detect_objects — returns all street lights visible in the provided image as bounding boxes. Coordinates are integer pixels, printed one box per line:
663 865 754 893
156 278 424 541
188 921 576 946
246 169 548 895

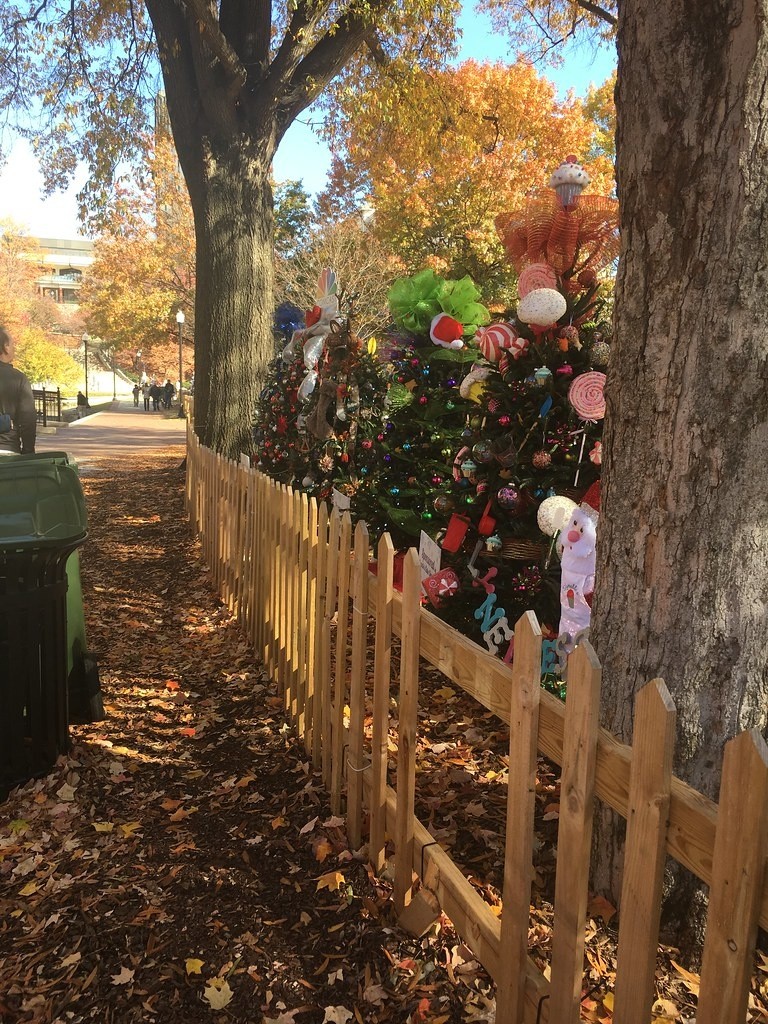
175 310 185 417
551 155 590 299
82 332 89 401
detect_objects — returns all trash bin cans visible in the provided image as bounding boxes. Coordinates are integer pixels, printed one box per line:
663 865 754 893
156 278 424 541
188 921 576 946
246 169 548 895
0 450 107 806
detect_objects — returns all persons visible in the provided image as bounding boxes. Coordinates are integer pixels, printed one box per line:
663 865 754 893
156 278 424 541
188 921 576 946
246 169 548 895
0 326 38 456
77 391 87 418
132 384 140 407
165 379 174 409
149 381 162 411
142 382 151 411
